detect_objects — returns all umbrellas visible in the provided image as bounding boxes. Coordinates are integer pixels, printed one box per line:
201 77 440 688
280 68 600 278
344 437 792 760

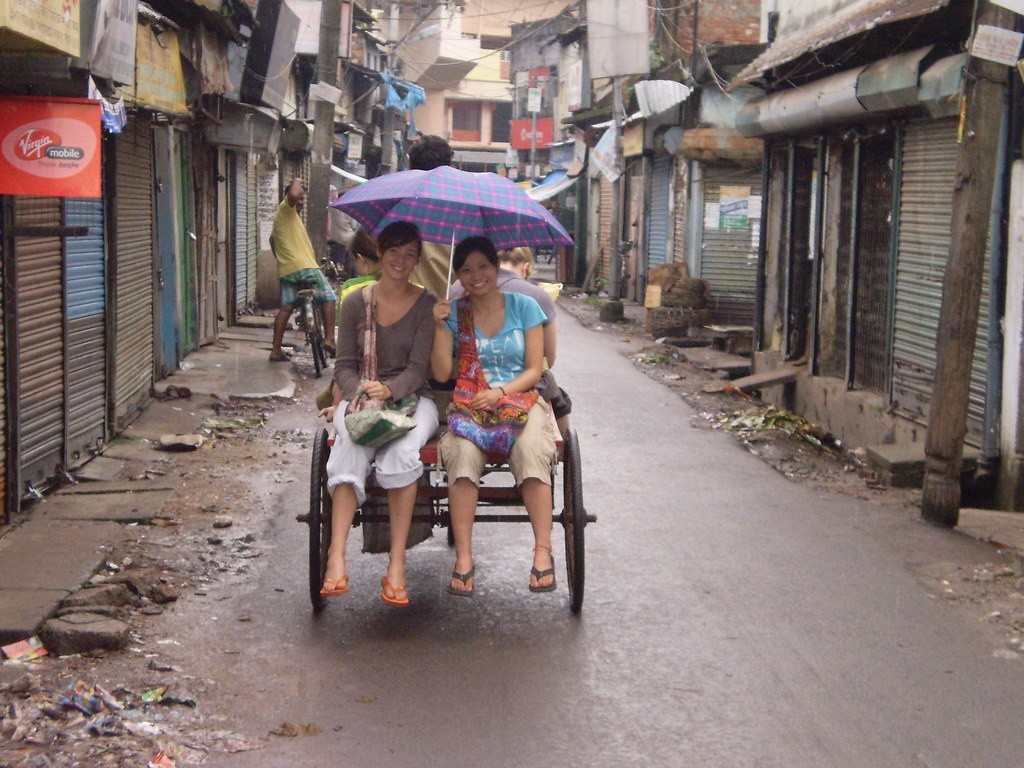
325 165 574 319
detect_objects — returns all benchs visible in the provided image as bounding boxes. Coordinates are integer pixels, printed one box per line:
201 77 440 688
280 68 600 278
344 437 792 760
327 361 563 452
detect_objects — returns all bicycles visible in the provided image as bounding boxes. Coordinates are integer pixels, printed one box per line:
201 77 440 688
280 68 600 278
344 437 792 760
295 257 339 380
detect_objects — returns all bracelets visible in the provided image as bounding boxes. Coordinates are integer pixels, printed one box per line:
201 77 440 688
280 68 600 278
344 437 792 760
498 387 505 395
333 402 337 403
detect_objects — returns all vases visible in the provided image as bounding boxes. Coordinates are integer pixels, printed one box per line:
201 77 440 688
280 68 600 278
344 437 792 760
644 284 700 309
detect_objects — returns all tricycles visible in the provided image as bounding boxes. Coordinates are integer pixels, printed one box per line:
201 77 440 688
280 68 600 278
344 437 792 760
297 369 598 619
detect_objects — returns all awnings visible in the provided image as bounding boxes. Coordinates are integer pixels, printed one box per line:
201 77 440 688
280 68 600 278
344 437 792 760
527 177 579 200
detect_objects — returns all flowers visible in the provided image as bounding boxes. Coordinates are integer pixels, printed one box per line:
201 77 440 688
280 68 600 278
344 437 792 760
649 263 711 300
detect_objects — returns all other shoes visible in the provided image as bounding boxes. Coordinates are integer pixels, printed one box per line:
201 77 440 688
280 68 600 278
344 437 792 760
269 352 290 361
320 341 335 359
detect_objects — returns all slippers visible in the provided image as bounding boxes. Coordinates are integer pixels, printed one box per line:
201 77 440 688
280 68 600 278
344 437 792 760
448 562 476 597
380 576 409 606
528 555 557 592
320 573 350 596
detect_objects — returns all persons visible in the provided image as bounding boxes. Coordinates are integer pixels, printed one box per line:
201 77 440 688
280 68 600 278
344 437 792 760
410 135 457 298
269 178 337 362
321 221 437 604
327 192 357 280
450 244 572 440
430 236 558 593
551 202 569 229
316 226 379 424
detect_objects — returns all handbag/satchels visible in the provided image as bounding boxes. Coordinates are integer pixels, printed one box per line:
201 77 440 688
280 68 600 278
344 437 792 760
343 390 416 448
446 379 538 458
